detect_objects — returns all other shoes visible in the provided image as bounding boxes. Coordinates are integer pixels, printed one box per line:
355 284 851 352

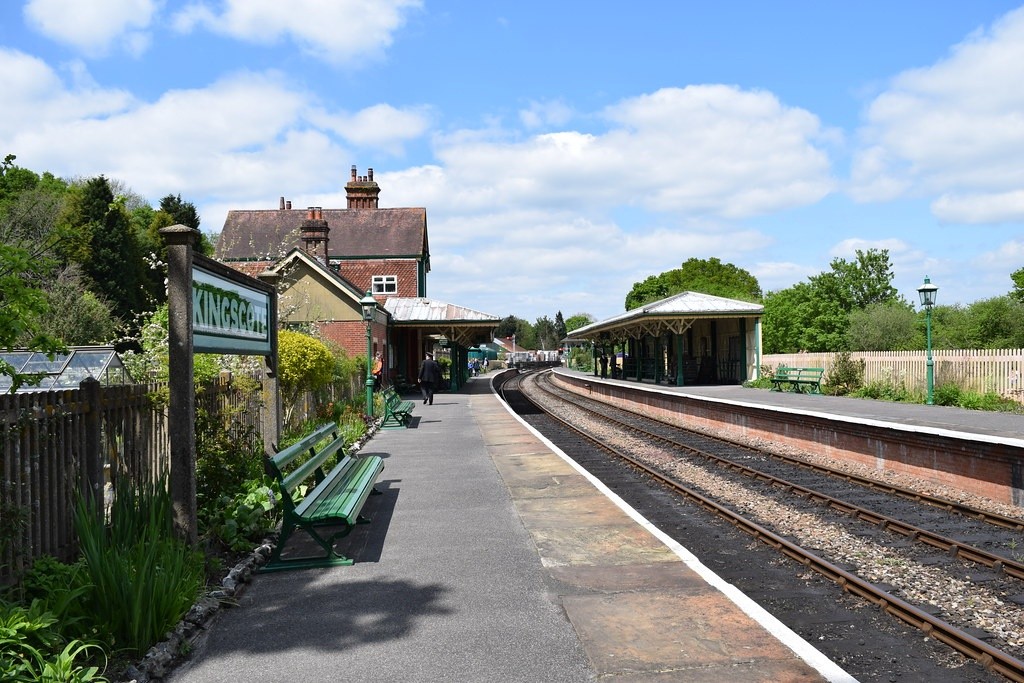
429 403 432 405
423 396 428 405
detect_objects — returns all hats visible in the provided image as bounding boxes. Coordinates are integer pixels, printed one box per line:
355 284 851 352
426 351 434 357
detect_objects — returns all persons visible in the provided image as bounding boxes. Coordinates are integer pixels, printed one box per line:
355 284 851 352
609 352 617 379
371 354 383 392
417 352 440 405
468 357 489 377
599 354 608 379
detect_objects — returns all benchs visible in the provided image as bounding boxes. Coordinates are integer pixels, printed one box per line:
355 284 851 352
379 385 415 429
770 367 824 394
257 421 384 572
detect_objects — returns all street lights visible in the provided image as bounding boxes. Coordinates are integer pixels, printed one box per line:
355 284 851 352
358 289 380 426
915 274 939 406
